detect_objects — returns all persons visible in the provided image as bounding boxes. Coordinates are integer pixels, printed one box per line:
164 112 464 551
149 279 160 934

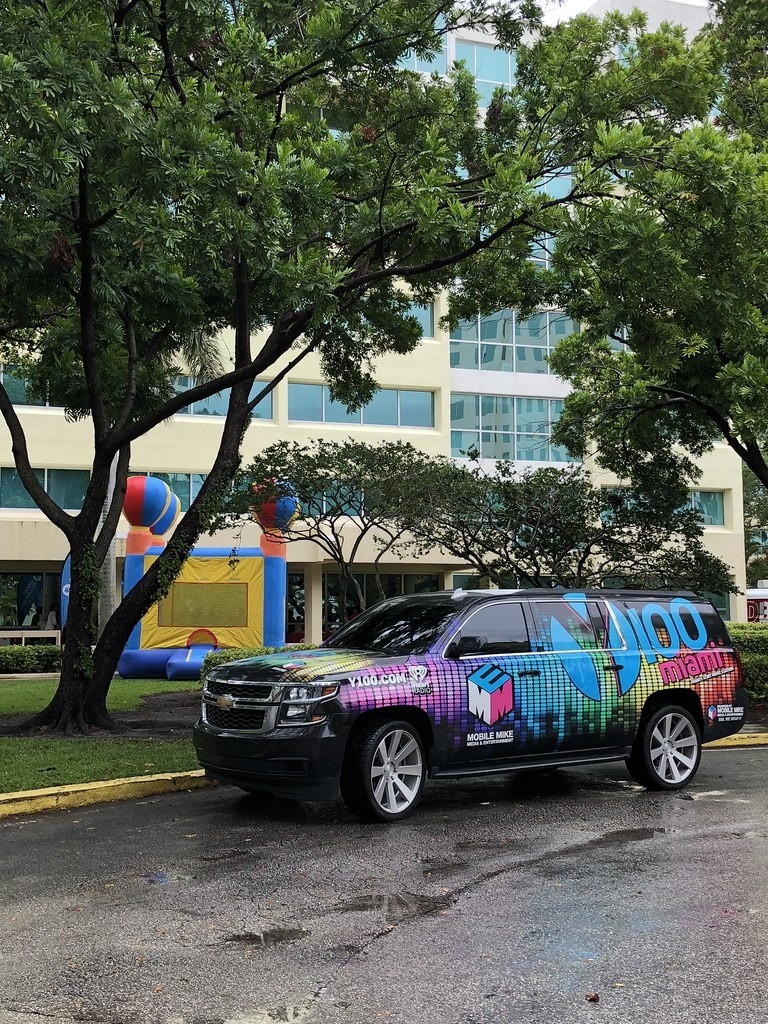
45 603 59 644
31 606 43 627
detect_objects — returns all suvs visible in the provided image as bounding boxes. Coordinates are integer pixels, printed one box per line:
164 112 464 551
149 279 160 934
192 586 750 824
